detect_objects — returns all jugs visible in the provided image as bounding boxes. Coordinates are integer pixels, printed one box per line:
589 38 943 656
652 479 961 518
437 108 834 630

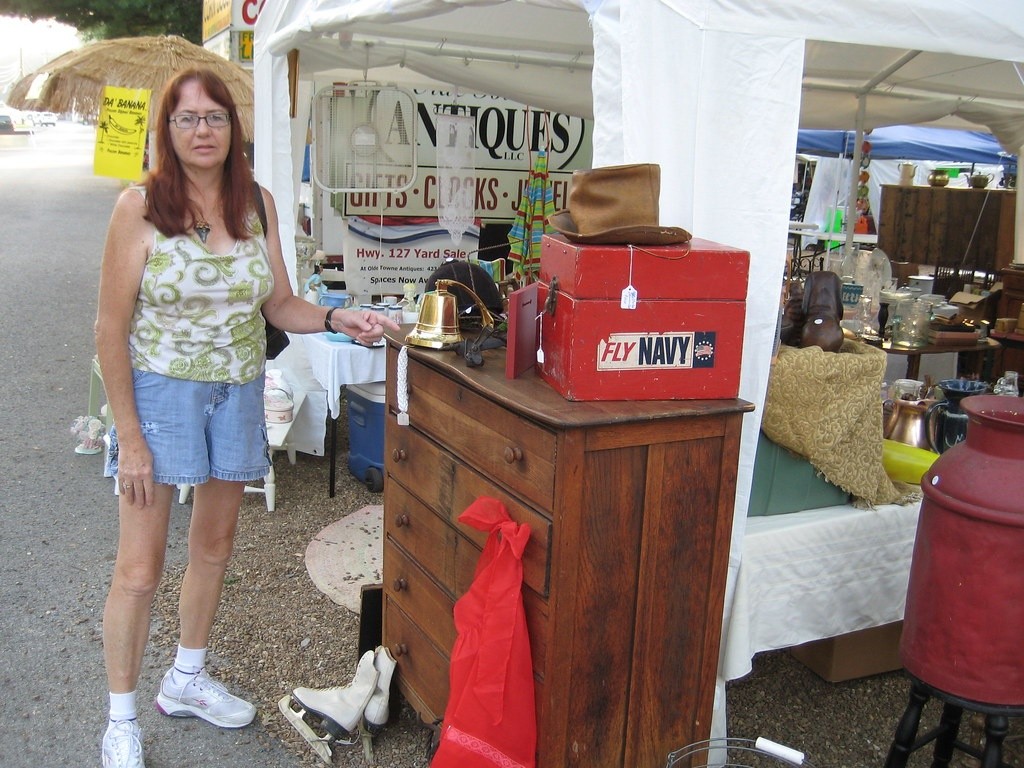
878 371 1019 457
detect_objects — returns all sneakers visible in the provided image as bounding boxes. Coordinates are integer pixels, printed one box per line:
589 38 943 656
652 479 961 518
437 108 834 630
155 664 257 729
102 718 146 768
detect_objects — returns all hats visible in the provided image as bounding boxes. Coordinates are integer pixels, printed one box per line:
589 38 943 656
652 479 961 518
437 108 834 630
547 163 692 246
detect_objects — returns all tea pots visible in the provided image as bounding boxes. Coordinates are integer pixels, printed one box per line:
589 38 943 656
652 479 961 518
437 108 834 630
898 160 918 187
964 171 995 189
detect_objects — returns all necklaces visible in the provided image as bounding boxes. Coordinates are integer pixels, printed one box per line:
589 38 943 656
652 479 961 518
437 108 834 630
191 200 220 243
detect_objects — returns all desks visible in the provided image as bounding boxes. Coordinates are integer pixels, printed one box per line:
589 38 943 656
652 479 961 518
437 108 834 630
855 332 1002 380
178 394 308 512
787 230 877 283
381 318 755 768
709 485 925 768
286 331 386 496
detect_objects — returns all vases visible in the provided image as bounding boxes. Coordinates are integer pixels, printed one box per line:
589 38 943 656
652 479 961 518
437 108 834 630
926 380 986 457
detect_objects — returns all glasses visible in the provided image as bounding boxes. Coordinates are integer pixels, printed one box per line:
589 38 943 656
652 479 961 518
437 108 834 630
168 113 230 129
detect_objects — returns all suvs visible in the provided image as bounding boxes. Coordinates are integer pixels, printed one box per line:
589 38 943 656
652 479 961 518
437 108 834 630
0 116 15 133
32 112 59 126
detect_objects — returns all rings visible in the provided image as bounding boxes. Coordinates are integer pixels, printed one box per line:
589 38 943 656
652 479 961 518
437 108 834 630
123 484 131 488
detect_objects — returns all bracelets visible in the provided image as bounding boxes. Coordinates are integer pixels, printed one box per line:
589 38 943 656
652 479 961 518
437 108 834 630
325 306 343 334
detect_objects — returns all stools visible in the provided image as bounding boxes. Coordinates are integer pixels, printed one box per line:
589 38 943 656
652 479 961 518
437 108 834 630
89 354 112 478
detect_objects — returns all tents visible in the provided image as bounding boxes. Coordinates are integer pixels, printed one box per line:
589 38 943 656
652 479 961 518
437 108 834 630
265 1 1024 276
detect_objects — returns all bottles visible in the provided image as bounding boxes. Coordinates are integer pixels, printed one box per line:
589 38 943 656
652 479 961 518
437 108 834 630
854 276 959 350
896 396 1024 707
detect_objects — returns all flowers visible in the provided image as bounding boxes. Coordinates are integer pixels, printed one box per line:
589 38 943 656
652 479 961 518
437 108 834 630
69 415 106 454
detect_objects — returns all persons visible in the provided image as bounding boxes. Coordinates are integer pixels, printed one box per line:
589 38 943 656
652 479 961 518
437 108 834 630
99 67 402 768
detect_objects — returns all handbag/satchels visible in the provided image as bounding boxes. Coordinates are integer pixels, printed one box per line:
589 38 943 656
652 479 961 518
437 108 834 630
250 179 290 361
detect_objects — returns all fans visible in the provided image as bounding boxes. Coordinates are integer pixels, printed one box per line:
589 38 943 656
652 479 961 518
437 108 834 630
312 85 418 193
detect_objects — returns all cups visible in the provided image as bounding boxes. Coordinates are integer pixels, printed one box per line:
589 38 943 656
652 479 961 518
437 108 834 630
928 168 949 186
360 297 403 324
994 318 1018 334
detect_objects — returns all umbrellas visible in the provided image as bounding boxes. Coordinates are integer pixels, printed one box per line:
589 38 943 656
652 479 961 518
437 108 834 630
1 34 262 171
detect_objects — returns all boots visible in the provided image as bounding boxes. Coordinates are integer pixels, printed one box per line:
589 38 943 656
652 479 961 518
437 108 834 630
358 645 397 763
278 650 380 764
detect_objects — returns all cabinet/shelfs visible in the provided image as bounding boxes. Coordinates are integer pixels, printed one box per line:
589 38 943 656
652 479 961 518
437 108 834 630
998 266 1024 324
879 184 1017 271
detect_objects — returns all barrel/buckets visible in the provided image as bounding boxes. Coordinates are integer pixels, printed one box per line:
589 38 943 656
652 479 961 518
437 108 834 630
263 386 295 447
319 292 352 308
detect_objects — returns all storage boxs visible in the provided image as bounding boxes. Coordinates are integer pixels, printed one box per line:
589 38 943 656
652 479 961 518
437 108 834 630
783 619 907 683
341 381 387 492
947 281 1004 325
537 234 748 400
